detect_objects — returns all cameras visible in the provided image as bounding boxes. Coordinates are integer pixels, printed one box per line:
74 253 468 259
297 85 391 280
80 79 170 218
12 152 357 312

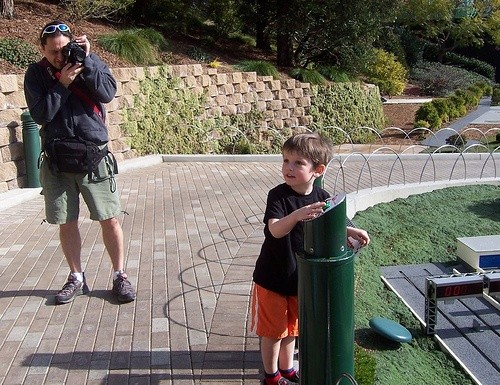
62 40 86 68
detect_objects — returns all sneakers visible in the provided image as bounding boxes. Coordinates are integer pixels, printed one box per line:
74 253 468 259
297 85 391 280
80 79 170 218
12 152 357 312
54 272 90 303
112 271 136 303
263 369 299 385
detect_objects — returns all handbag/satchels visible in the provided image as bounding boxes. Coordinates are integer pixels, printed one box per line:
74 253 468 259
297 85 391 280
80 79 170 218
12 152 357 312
51 136 100 173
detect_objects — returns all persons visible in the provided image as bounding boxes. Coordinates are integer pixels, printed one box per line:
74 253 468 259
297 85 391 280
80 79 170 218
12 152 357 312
23 19 137 304
250 132 370 385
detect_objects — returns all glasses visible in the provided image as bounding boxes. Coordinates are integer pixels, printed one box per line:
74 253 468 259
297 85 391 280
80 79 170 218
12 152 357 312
41 23 71 38
